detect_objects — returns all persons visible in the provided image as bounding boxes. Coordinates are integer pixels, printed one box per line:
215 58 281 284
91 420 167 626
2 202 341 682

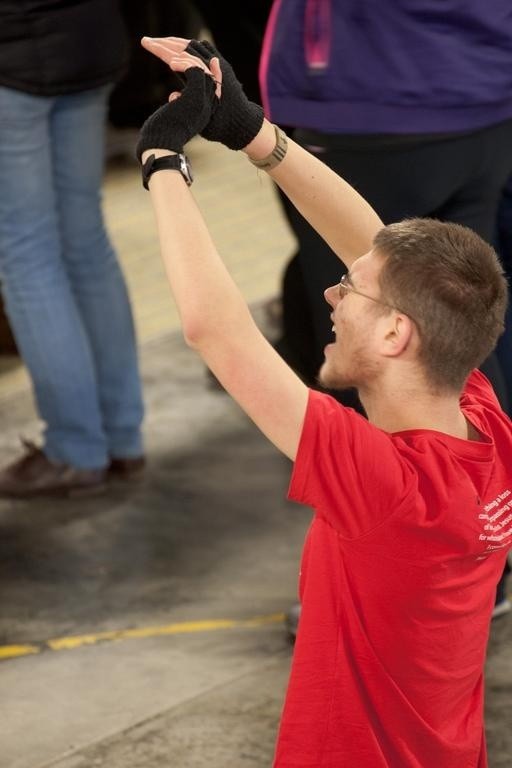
0 1 170 501
211 283 292 398
205 0 512 646
105 0 207 169
135 34 512 765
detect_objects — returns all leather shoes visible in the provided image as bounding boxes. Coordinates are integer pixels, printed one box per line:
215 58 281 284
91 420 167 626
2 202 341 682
1 442 143 500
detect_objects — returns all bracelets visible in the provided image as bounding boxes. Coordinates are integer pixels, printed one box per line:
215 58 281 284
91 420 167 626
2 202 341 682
247 121 287 172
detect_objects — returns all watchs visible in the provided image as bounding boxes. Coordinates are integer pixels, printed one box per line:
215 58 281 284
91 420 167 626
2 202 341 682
140 152 193 190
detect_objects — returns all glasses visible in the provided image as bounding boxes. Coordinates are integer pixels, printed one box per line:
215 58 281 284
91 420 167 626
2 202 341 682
339 274 423 337
